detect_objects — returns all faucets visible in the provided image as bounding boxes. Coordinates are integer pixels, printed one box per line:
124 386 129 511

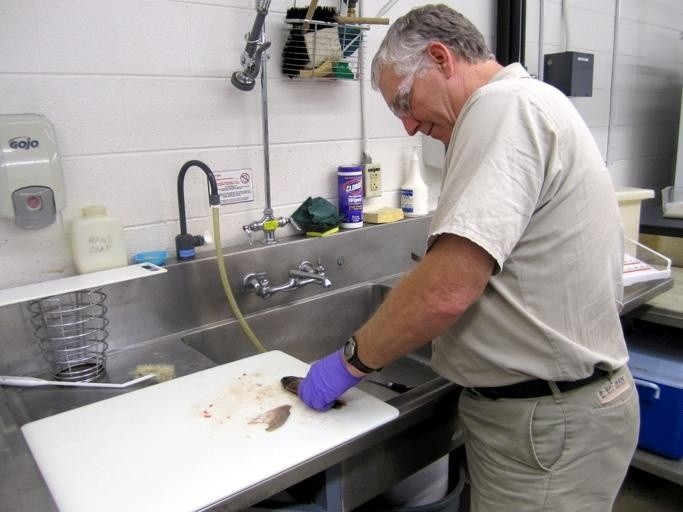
289 270 332 289
174 159 220 260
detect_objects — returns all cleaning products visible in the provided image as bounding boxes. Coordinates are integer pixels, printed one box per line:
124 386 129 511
400 144 428 217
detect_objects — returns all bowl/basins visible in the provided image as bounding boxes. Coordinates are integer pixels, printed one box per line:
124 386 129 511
401 458 467 511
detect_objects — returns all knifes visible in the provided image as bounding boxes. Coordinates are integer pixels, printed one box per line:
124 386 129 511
363 369 416 394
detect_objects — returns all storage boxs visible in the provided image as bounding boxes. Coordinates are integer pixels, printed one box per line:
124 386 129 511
623 327 683 460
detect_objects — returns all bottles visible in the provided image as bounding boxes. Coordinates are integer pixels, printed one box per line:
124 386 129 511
337 164 364 230
71 203 128 275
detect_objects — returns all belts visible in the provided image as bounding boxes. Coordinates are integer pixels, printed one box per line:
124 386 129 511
465 365 620 400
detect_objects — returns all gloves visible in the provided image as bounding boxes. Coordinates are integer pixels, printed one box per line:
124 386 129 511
296 349 366 413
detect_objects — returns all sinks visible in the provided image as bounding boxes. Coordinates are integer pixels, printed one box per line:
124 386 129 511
181 284 442 402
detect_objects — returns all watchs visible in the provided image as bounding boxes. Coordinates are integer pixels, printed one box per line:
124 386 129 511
341 334 385 374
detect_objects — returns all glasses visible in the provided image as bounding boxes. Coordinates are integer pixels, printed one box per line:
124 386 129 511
389 48 429 117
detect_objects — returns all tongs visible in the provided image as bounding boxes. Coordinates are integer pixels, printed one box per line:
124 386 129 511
22 347 400 512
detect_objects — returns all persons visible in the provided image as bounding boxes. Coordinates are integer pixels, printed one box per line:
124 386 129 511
297 2 643 512
